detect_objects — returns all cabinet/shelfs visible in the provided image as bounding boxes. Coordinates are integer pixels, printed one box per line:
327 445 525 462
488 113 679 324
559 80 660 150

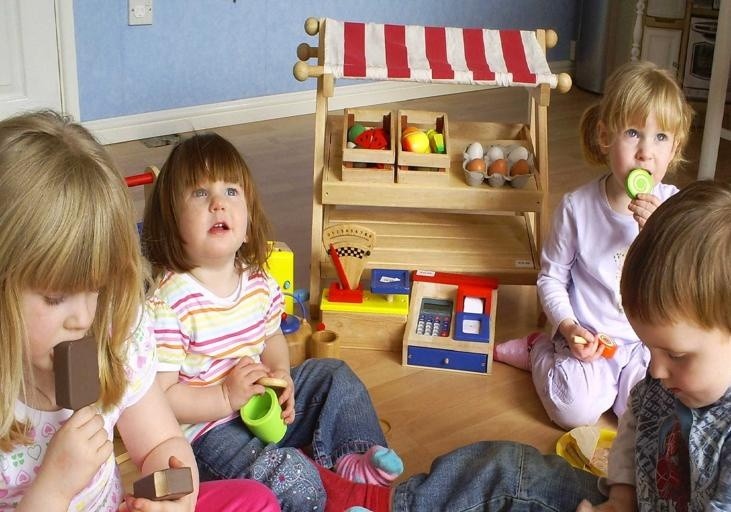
603 0 731 102
294 19 572 327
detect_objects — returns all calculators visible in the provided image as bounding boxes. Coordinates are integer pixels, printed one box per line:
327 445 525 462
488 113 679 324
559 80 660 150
416 298 452 337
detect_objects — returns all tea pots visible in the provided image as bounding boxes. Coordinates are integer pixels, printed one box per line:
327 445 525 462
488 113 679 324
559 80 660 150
277 292 325 369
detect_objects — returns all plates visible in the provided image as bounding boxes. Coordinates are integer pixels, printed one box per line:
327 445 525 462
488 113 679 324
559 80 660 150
555 427 619 476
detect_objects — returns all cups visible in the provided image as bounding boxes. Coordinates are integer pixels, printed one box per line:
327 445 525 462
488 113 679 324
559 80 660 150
311 329 341 359
240 387 287 444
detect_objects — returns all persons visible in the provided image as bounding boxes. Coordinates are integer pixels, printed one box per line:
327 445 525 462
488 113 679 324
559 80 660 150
494 58 696 431
1 107 278 511
126 129 406 510
390 178 731 509
